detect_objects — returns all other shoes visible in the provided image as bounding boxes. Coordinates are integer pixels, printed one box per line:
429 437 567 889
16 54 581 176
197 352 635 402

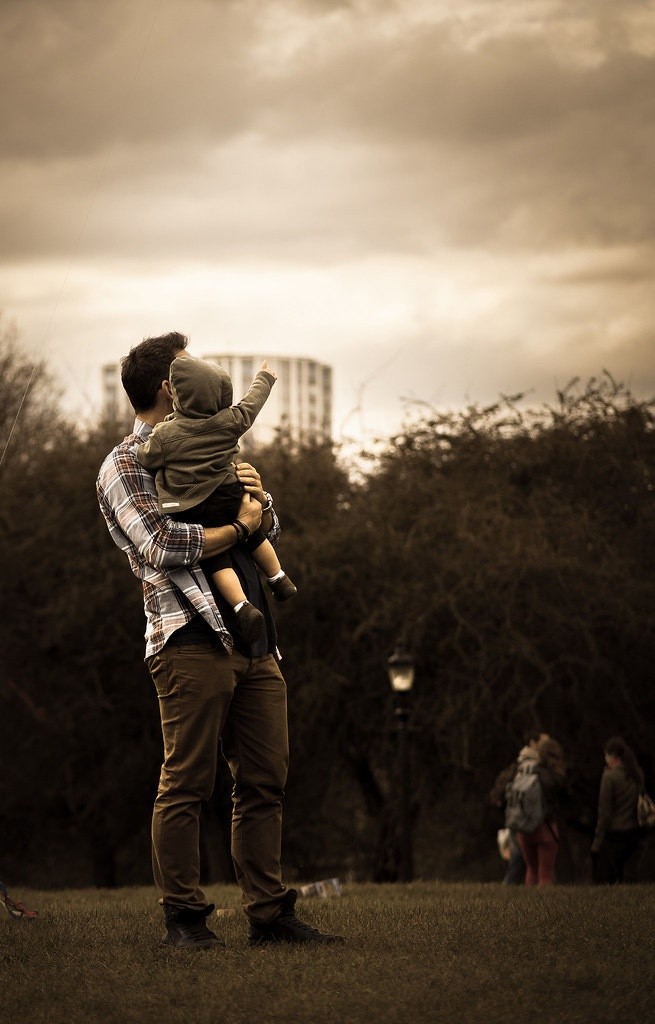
237 601 264 646
269 574 298 604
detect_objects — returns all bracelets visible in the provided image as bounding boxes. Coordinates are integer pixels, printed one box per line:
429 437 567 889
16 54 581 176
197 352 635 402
230 519 252 545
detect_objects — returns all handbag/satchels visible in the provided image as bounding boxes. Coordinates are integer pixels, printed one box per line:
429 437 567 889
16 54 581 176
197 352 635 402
497 828 518 860
638 789 655 827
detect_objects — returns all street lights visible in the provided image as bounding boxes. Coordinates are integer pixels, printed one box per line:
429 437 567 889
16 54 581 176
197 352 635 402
386 644 418 884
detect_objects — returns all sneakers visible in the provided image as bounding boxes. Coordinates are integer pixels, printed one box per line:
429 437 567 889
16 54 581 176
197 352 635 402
164 903 226 953
248 888 345 949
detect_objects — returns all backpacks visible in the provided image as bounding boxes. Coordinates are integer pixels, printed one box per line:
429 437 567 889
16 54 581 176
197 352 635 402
506 771 545 834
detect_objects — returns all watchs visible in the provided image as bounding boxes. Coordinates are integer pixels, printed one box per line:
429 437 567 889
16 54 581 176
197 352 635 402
262 491 274 515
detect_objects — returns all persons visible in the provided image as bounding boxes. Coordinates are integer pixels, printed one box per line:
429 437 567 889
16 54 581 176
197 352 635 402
95 332 345 950
137 354 298 645
591 736 646 886
491 731 565 886
